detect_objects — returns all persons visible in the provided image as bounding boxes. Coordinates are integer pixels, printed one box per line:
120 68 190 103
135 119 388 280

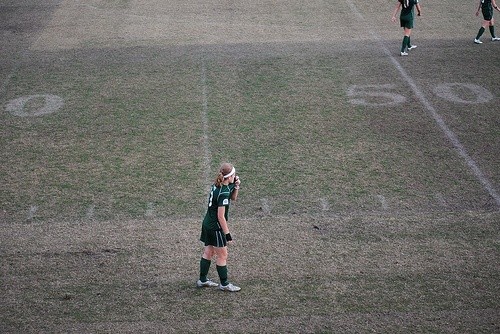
474 0 500 44
196 162 241 292
391 0 421 56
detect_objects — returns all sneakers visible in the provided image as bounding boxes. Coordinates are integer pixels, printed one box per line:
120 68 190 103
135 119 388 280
492 36 500 41
407 45 416 51
400 51 408 55
475 39 483 43
220 283 241 292
197 280 218 286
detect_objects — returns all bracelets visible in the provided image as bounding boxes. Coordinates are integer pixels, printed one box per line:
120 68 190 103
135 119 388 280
234 187 240 189
